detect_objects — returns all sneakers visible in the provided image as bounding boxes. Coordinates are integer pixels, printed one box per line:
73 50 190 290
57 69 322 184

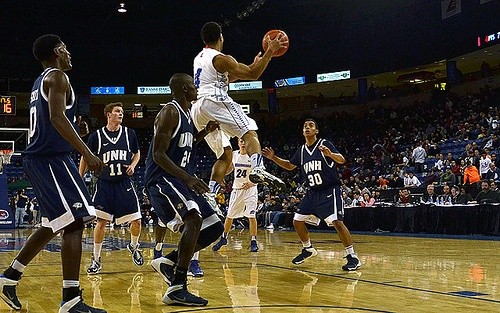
162 284 208 306
249 241 258 251
154 248 162 259
59 288 106 313
127 242 144 266
292 247 317 264
87 257 101 274
212 236 227 251
0 275 21 311
187 259 204 276
150 257 175 285
342 254 361 271
202 191 224 219
249 167 286 192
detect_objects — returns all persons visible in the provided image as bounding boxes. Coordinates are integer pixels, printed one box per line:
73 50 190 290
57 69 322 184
145 73 220 307
79 102 144 275
212 139 258 252
0 85 500 204
262 119 361 271
191 22 286 197
0 34 106 313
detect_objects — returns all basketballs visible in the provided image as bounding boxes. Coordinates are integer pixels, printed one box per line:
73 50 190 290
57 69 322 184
262 29 289 56
